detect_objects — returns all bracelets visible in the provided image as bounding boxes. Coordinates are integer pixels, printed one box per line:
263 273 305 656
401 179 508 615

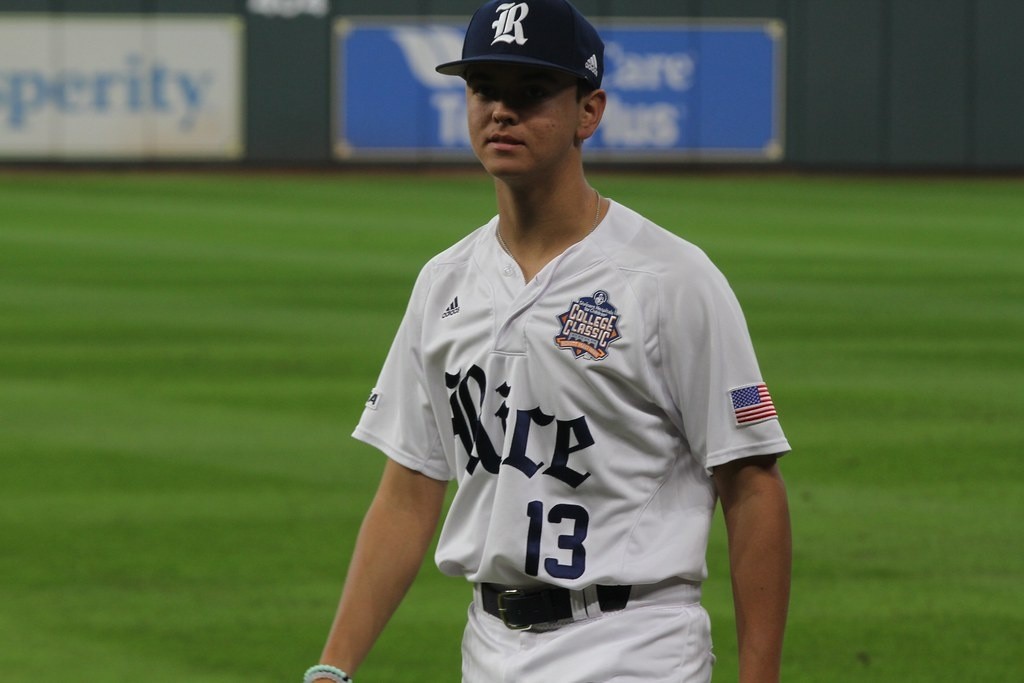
303 663 353 683
302 0 795 683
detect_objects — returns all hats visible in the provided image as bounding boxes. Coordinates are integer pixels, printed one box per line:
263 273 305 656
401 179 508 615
435 0 605 89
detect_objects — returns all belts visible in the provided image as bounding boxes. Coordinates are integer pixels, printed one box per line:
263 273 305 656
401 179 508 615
481 583 632 630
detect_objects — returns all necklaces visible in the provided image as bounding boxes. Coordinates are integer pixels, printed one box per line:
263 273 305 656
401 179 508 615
496 189 601 261
